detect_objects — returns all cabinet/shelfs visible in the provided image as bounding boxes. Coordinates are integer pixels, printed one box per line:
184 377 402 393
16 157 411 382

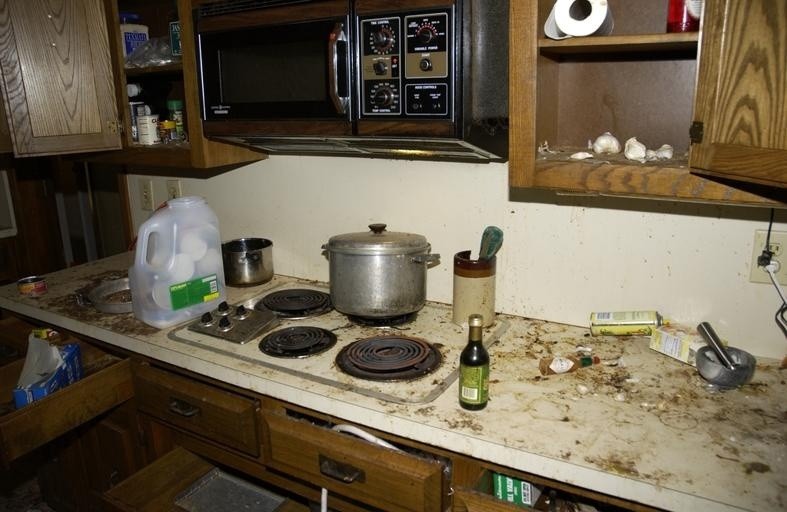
131 360 262 470
0 0 269 168
261 399 449 512
508 0 787 210
1 311 132 510
456 458 632 509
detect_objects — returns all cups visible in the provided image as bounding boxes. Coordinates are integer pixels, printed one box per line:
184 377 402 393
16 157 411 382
454 250 497 329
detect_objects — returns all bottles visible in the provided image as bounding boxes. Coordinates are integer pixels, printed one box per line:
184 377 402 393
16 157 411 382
459 314 491 410
166 88 185 134
588 309 674 337
667 0 701 31
166 0 184 66
118 13 149 56
539 355 602 374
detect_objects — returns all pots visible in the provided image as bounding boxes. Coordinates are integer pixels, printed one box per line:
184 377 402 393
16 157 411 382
321 222 439 319
220 238 274 286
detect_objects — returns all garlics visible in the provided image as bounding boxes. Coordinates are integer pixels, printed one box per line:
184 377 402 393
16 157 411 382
625 136 673 161
593 132 620 153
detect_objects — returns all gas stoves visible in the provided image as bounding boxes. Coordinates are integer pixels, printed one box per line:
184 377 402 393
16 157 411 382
167 276 512 406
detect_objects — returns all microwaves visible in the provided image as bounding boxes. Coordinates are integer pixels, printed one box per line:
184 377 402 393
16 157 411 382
191 0 510 121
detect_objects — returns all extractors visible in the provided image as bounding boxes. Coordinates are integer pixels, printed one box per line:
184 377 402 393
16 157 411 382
205 124 510 165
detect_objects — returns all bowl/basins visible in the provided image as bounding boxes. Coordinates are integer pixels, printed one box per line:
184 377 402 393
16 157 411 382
698 347 756 388
87 278 134 315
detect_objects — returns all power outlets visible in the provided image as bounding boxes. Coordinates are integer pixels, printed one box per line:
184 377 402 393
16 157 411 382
749 231 786 286
138 179 154 210
167 180 181 201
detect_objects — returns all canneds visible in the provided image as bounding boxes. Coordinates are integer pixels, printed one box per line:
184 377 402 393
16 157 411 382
159 120 177 145
17 276 48 296
